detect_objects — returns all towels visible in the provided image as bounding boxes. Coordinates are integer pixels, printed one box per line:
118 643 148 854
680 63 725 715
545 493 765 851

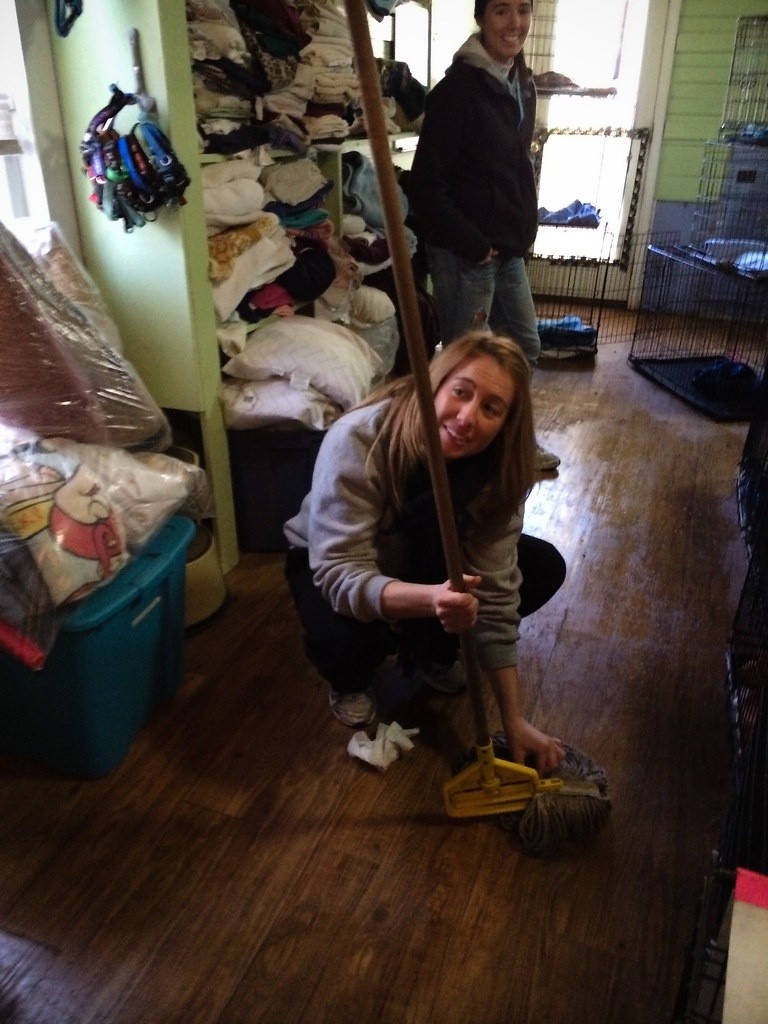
196 159 265 224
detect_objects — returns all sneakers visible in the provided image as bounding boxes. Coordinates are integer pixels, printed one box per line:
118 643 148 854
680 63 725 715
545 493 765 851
533 443 561 472
328 686 377 727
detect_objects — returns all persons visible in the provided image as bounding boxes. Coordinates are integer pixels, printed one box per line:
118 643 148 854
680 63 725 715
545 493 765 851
282 329 567 779
405 1 562 473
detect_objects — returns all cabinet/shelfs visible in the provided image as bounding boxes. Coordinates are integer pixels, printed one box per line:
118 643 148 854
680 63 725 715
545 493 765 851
200 124 420 357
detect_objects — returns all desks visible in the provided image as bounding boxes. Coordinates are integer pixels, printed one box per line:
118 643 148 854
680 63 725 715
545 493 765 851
692 867 768 1024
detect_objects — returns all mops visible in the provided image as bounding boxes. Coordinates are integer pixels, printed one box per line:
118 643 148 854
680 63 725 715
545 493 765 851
343 0 613 859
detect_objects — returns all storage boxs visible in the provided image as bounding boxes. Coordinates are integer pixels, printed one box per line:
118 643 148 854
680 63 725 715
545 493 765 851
36 514 198 777
